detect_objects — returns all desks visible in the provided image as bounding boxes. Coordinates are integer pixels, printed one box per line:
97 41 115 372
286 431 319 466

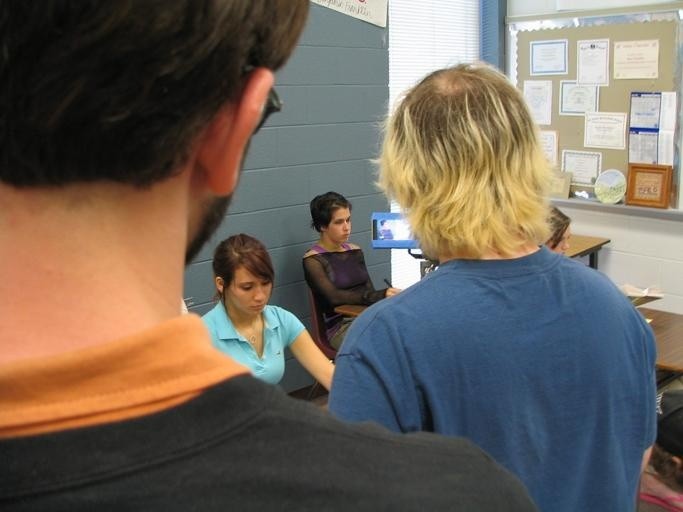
562 233 683 431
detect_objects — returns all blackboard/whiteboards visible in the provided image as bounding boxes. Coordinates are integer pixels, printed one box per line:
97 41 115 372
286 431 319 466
504 2 683 221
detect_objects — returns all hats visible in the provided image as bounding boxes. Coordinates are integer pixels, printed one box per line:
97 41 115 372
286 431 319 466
656 388 683 458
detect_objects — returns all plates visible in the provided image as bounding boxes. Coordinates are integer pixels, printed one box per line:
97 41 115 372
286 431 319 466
595 169 627 204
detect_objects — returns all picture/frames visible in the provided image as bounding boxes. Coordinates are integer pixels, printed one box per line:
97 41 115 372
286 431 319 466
625 162 673 209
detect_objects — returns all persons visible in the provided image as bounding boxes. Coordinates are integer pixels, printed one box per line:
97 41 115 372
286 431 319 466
328 61 658 512
639 388 683 510
0 0 540 512
303 191 402 352
201 233 335 392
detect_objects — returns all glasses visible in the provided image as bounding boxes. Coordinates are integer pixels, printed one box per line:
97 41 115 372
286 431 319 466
232 62 282 136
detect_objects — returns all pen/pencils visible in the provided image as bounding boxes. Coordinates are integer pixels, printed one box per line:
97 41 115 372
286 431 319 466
384 279 393 288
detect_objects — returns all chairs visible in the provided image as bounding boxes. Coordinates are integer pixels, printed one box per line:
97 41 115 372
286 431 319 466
304 284 339 403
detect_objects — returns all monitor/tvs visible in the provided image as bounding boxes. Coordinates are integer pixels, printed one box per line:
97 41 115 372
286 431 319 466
371 213 420 249
